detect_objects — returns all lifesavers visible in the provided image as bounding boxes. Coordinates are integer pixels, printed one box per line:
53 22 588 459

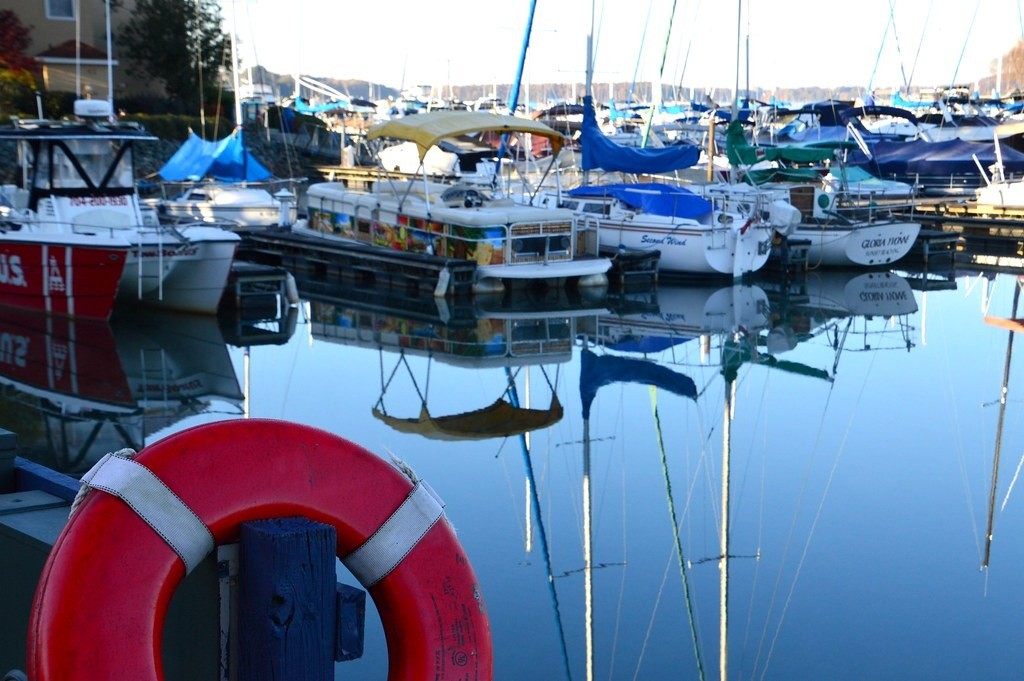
25 417 493 681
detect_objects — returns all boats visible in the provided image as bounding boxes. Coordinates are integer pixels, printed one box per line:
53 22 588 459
0 303 255 501
224 295 300 353
0 3 1024 317
762 266 922 386
284 268 609 441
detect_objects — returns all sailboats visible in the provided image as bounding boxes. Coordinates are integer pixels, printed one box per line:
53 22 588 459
578 275 773 681
